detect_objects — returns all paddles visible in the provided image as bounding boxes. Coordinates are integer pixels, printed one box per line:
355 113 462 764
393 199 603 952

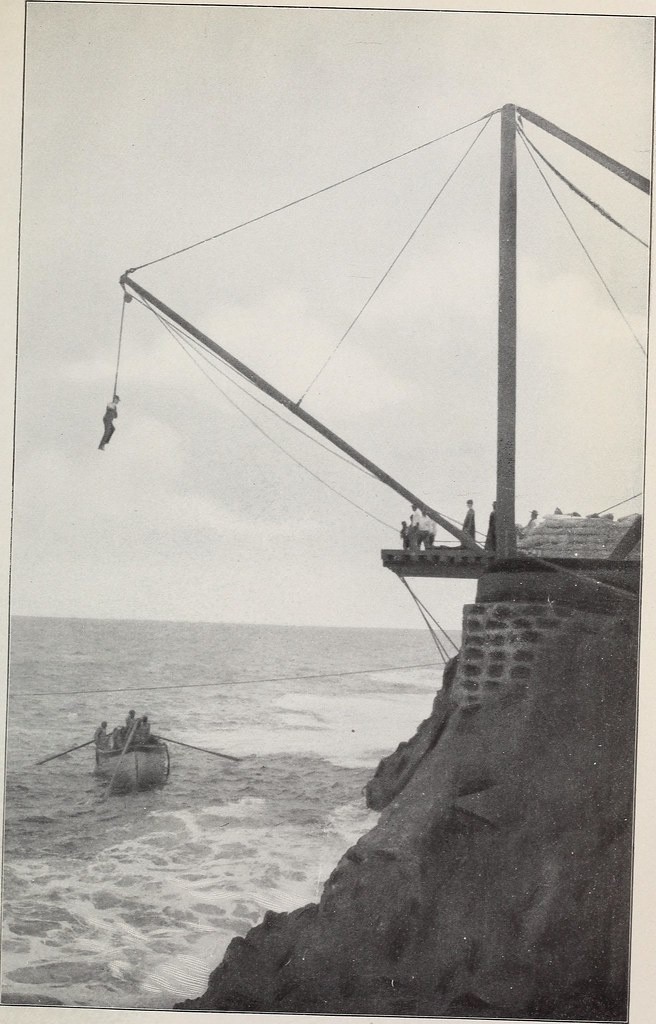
24 737 97 769
101 718 142 797
150 732 243 762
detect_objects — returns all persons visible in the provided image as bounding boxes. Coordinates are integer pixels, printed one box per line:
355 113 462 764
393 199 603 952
94 710 151 751
522 509 540 528
400 504 437 550
484 501 497 552
462 500 475 541
98 395 121 451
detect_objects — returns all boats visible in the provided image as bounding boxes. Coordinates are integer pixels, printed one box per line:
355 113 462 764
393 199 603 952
94 717 170 798
